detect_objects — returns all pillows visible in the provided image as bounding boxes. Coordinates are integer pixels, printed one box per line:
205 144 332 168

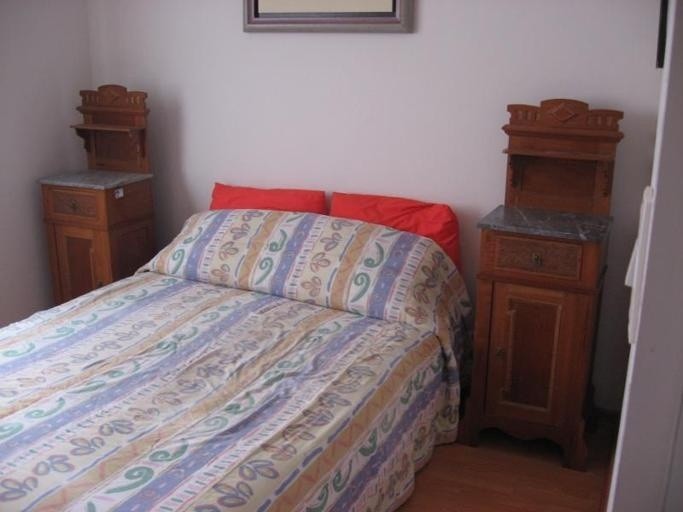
210 182 462 273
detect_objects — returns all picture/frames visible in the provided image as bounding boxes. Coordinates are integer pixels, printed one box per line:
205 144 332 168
241 0 414 34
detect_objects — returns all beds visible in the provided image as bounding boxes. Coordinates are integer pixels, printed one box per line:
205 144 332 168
0 211 473 512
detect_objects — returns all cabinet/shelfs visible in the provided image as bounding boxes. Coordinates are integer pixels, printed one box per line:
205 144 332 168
458 98 627 474
34 84 159 306
